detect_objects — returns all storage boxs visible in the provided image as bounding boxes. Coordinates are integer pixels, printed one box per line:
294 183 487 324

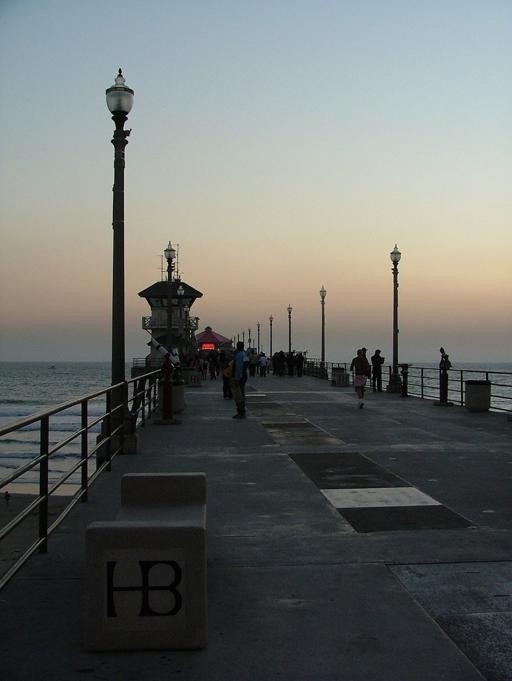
87 470 207 651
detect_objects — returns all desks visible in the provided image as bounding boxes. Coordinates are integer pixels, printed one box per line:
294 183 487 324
164 241 185 352
105 67 134 381
233 328 250 347
256 321 260 354
287 304 292 352
389 243 402 373
268 314 273 356
319 285 326 361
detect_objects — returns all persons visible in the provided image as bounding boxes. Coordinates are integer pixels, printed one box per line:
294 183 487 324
369 350 384 395
355 348 370 393
228 342 248 421
221 349 232 401
350 349 370 410
184 348 308 379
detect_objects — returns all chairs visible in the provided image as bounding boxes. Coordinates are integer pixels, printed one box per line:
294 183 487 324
231 410 247 419
358 401 364 409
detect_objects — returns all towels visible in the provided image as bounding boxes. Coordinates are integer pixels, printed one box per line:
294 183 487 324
159 380 185 414
332 367 344 386
465 380 491 412
182 367 198 385
